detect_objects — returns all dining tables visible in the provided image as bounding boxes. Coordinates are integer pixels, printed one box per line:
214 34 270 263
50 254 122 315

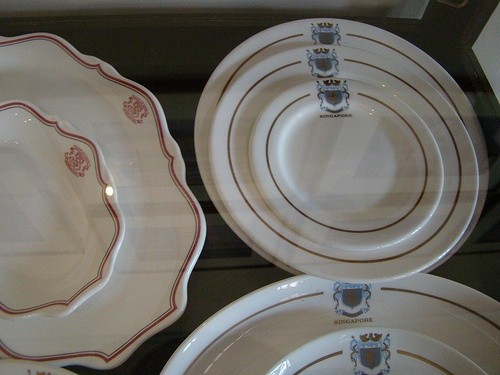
0 4 500 375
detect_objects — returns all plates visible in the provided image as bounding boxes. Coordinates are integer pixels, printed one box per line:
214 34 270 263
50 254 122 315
1 32 207 370
0 100 126 319
263 326 491 375
154 275 500 375
193 14 489 284
